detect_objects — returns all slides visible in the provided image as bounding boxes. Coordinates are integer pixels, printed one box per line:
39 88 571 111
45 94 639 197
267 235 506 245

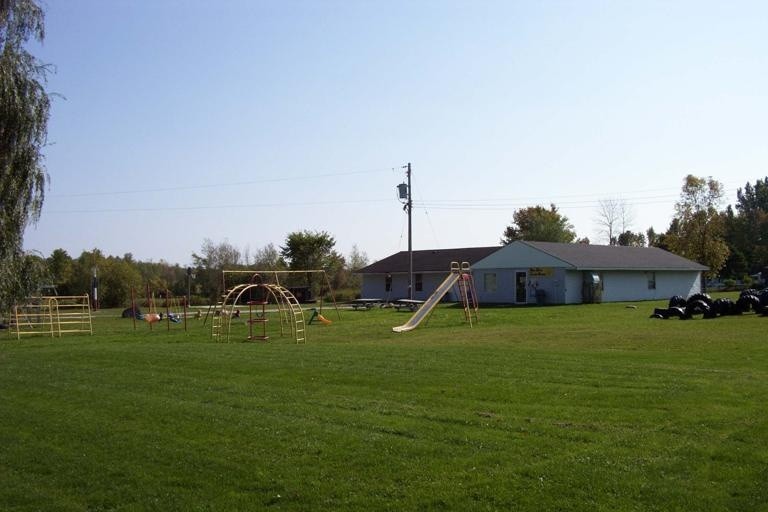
391 274 460 333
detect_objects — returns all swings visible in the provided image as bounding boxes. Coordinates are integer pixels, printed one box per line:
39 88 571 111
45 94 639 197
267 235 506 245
134 285 183 326
